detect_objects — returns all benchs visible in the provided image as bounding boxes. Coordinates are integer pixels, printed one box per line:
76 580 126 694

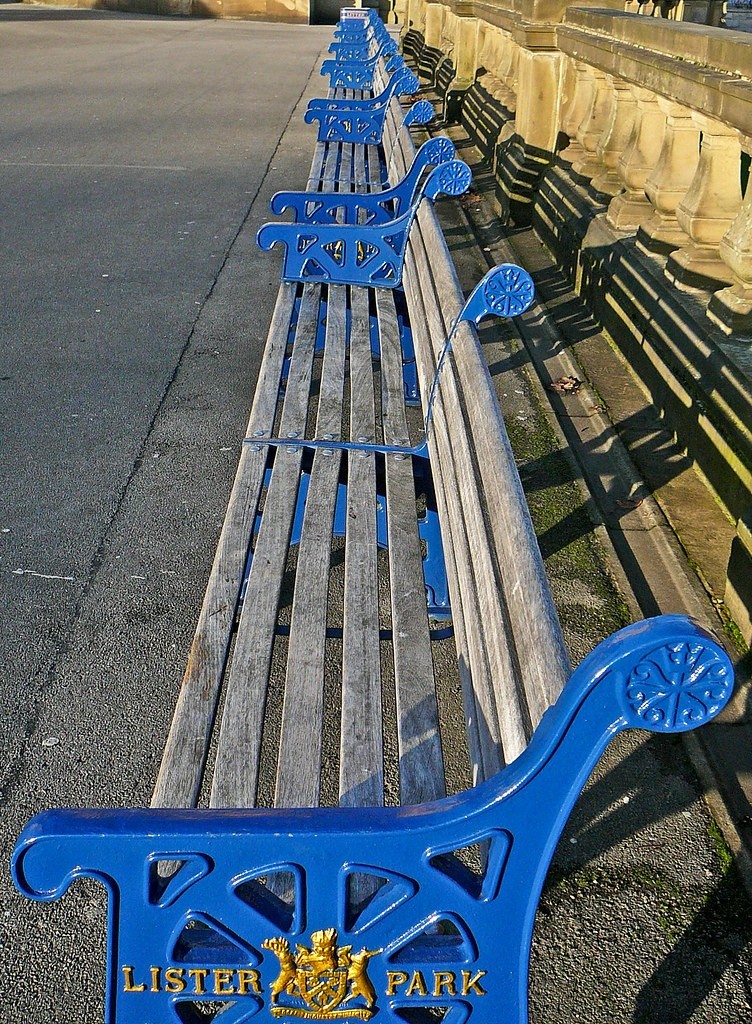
11 8 735 1024
